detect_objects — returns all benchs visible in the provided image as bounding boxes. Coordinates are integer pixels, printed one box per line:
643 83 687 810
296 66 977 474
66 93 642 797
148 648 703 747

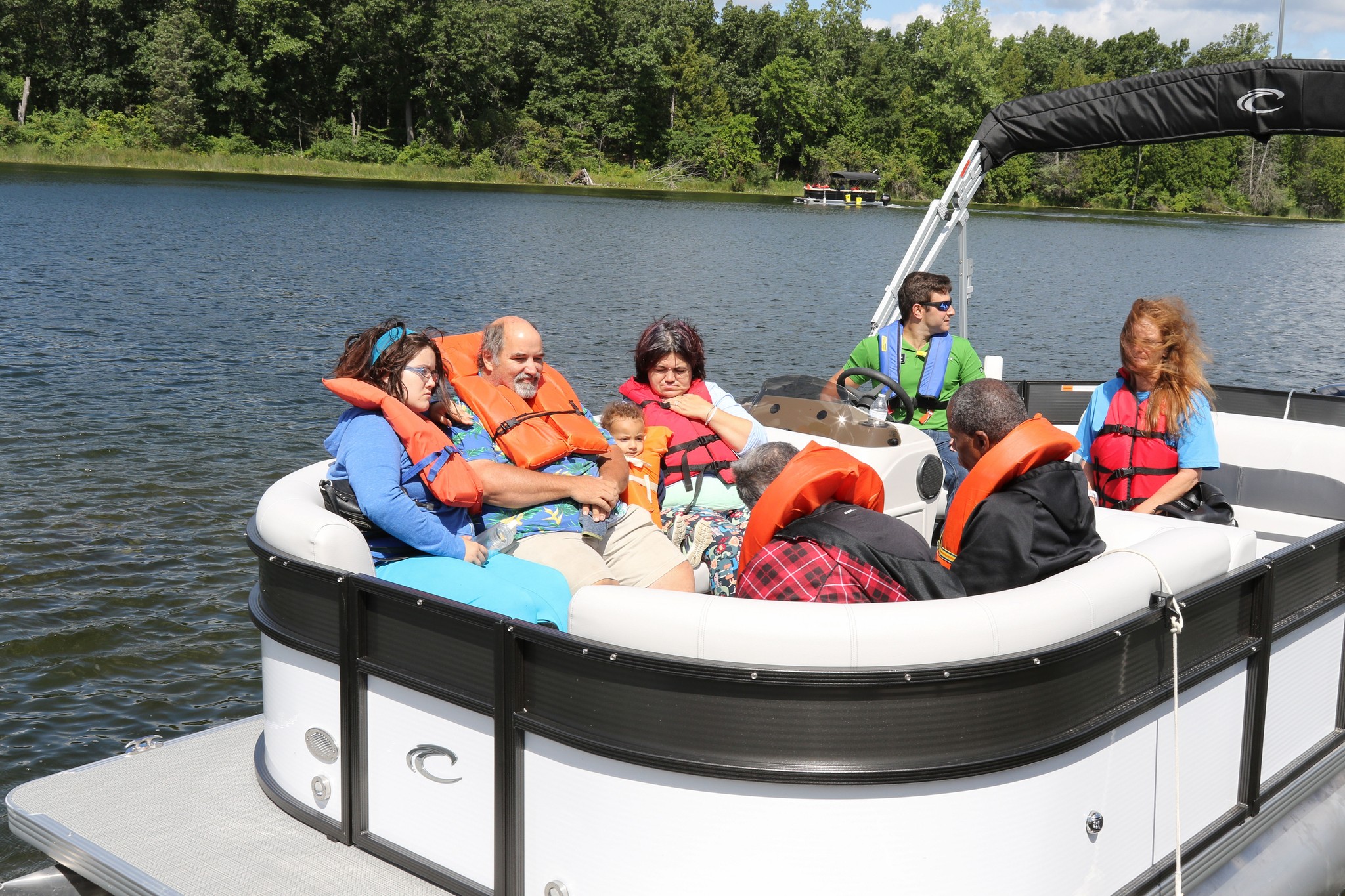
252 409 1345 658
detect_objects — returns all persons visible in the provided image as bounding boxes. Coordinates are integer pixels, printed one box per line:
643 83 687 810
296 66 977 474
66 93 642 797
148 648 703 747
819 272 986 536
729 441 966 604
933 377 1107 596
619 314 768 597
322 319 572 633
599 399 713 570
1073 296 1220 515
451 315 696 594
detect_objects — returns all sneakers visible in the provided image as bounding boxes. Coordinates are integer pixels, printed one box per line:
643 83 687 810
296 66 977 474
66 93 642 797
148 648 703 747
680 519 712 569
663 515 686 553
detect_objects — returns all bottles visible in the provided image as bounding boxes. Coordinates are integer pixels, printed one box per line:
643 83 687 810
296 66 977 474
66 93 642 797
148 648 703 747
867 393 888 427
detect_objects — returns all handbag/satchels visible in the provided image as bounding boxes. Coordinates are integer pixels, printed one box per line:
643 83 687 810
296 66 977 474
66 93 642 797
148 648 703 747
1154 482 1239 528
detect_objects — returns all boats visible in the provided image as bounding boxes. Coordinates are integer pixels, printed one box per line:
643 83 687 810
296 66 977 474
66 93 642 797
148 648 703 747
793 168 882 204
1 55 1340 895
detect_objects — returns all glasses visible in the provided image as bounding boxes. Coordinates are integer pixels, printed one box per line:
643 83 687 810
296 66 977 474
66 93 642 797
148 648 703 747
403 365 440 384
911 296 952 312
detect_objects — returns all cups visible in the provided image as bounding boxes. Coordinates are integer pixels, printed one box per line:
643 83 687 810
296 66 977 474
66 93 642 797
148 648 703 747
470 519 520 557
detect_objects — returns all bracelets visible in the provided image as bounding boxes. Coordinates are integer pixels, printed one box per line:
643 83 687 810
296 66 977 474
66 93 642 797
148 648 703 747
1087 490 1099 507
705 406 717 425
706 406 717 423
